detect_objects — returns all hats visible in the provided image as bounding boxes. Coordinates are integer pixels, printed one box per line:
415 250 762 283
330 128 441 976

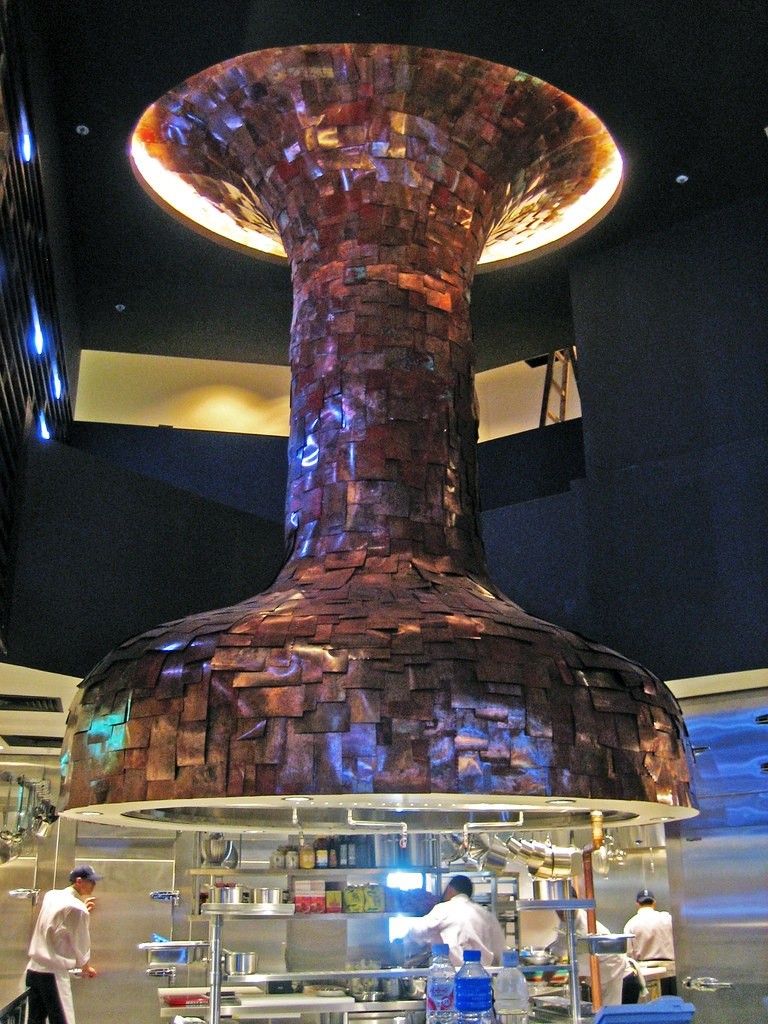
637 890 654 902
70 865 100 880
449 875 473 897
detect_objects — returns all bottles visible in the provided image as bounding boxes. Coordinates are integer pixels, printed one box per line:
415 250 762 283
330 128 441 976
494 951 530 1024
454 950 496 1024
271 834 357 870
425 943 459 1024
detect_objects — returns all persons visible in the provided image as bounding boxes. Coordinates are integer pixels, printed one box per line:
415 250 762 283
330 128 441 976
623 889 677 995
21 864 105 1024
408 874 507 966
540 886 648 1012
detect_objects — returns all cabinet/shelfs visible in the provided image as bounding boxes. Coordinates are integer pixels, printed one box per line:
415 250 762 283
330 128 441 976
187 867 519 981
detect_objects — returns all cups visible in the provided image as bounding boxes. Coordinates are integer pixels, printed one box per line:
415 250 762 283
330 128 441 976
212 886 282 906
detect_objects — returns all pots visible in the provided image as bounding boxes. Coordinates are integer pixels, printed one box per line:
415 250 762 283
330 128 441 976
578 934 636 955
346 961 427 1003
0 770 55 865
459 821 625 881
224 951 259 976
148 948 187 964
524 953 553 967
